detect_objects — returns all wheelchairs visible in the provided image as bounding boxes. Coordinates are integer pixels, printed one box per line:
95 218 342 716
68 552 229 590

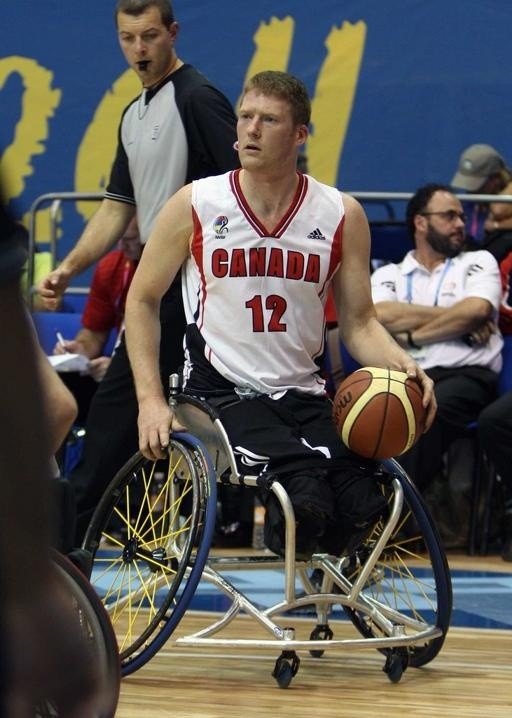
72 328 455 687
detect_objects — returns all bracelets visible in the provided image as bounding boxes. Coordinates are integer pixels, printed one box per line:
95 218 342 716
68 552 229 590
406 330 422 351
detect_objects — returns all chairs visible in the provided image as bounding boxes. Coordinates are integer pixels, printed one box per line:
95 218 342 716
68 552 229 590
26 308 122 477
385 332 512 556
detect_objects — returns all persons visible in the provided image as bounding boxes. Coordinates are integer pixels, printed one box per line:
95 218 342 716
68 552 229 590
53 214 143 384
478 250 512 562
0 171 79 542
450 142 512 242
36 1 386 618
370 181 503 501
125 70 439 559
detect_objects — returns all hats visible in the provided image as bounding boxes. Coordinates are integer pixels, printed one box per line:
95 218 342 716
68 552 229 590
449 143 498 192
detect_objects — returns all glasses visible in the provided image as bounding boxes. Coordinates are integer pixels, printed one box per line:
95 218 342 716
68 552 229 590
422 212 468 224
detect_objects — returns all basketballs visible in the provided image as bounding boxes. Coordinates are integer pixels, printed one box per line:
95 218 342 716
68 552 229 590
332 365 424 460
